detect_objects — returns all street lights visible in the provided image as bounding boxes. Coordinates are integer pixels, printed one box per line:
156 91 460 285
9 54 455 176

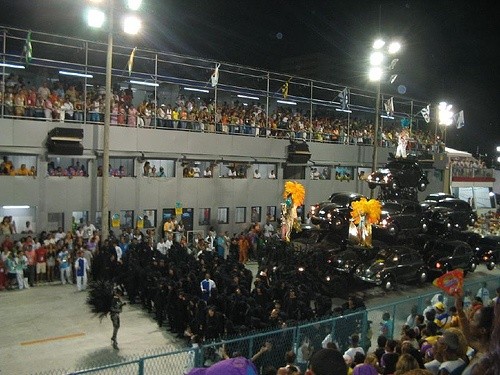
367 37 402 201
82 0 144 251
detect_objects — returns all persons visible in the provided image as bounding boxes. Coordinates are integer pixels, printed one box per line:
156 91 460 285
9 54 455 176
0 72 500 375
74 252 90 290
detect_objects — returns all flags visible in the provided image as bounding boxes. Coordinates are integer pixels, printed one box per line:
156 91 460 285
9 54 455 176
281 83 288 98
24 33 31 61
453 111 465 128
338 89 348 109
209 67 220 87
126 51 136 76
384 97 394 116
419 105 430 123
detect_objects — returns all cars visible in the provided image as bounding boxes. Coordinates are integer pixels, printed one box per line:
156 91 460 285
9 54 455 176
294 158 500 290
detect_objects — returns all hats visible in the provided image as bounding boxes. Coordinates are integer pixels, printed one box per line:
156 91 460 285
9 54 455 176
188 357 257 375
352 364 378 375
434 302 444 309
